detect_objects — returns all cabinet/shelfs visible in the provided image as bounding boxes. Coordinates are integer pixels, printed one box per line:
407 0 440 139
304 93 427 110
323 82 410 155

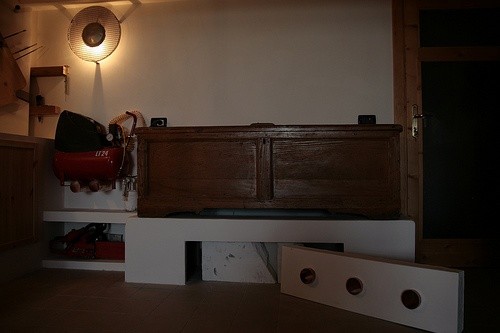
29 65 69 136
39 209 137 271
0 132 64 246
124 216 416 285
133 123 403 219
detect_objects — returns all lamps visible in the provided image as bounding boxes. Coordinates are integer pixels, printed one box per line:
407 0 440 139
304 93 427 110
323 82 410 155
66 6 122 65
